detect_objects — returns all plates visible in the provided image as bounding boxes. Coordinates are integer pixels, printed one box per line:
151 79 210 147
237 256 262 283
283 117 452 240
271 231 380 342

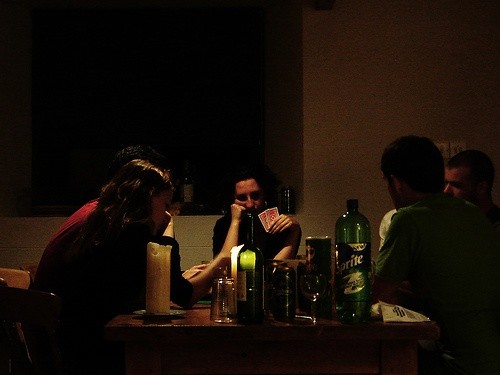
132 310 186 316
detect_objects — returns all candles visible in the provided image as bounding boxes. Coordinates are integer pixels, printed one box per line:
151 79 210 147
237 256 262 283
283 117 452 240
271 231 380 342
146 241 172 314
230 244 245 313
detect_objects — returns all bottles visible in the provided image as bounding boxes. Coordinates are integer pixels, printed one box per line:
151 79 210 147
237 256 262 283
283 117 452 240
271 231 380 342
210 266 234 323
334 198 372 324
283 184 296 214
179 161 195 215
237 213 264 323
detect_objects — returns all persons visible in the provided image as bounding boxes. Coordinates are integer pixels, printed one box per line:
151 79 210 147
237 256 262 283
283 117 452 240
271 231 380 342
24 146 302 375
372 135 500 375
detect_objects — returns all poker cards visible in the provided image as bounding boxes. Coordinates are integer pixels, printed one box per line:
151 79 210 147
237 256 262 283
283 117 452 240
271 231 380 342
258 206 279 232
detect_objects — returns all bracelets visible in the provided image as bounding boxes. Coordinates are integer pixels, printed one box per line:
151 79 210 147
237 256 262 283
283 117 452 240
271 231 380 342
286 240 298 244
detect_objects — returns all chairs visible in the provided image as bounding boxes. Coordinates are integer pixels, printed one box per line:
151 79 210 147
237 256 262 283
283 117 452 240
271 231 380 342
0 269 60 375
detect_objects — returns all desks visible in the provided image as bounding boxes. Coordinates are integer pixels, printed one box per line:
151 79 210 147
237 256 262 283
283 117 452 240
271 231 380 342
106 297 440 375
